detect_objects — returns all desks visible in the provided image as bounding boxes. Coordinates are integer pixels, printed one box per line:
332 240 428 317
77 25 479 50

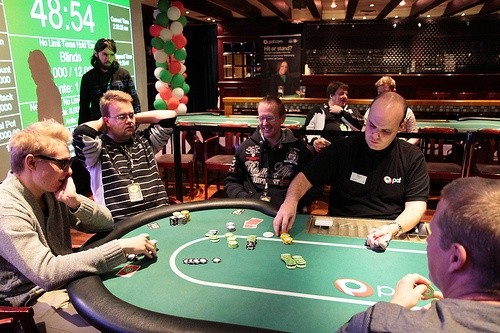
68 201 441 333
171 116 306 203
395 117 500 189
224 98 500 117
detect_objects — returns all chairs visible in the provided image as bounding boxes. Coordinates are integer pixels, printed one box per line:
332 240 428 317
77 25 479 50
156 109 247 199
415 126 500 200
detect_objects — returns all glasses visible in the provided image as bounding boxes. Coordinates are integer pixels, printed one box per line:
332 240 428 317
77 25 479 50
34 155 73 169
257 115 280 123
108 114 136 122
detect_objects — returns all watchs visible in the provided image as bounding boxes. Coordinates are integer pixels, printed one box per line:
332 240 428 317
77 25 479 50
391 222 403 237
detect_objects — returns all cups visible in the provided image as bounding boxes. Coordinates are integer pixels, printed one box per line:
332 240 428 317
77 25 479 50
278 86 284 98
300 86 306 98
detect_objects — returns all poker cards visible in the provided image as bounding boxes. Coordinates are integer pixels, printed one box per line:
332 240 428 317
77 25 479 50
371 225 391 244
115 263 141 278
243 218 263 228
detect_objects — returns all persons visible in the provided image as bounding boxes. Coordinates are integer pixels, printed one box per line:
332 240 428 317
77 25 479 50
273 89 429 251
78 37 141 125
72 89 177 223
264 60 300 98
224 95 320 212
361 76 419 144
334 175 500 333
302 81 364 155
0 116 158 333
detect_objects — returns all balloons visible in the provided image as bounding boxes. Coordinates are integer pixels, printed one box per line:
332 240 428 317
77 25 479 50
150 0 187 74
154 67 190 113
